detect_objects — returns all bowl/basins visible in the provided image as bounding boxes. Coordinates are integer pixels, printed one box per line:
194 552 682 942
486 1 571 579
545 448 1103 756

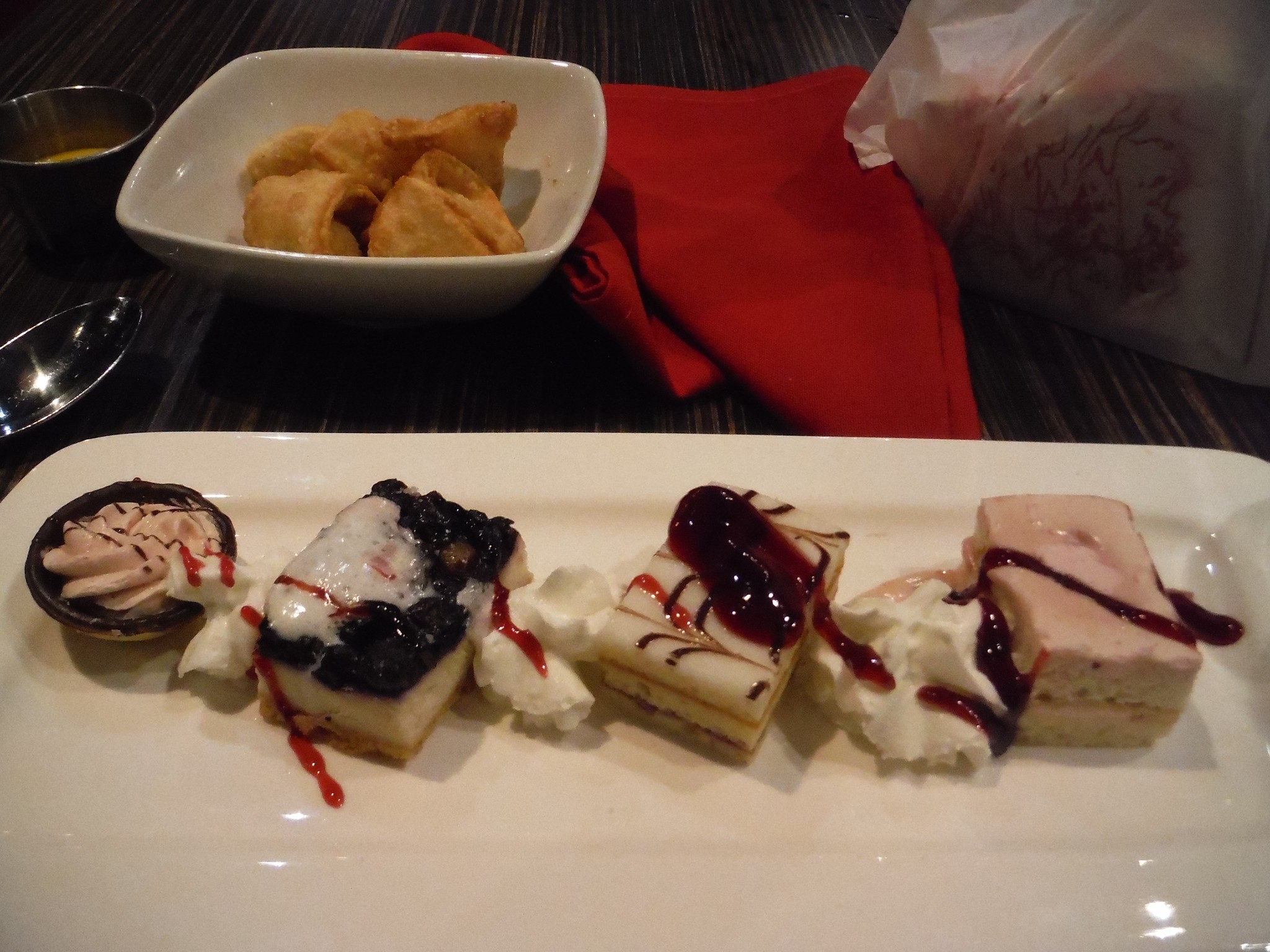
118 42 609 317
3 87 154 236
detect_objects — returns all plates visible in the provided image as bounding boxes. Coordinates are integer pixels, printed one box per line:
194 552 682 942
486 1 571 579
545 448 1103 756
0 416 1270 946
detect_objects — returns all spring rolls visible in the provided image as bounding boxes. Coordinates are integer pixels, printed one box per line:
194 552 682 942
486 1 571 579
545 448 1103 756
242 101 525 257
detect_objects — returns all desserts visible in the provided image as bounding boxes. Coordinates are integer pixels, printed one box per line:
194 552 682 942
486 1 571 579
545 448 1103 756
599 484 850 764
259 478 530 763
956 493 1207 748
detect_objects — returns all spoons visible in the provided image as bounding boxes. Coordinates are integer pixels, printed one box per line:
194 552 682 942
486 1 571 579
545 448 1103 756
1 294 142 442
